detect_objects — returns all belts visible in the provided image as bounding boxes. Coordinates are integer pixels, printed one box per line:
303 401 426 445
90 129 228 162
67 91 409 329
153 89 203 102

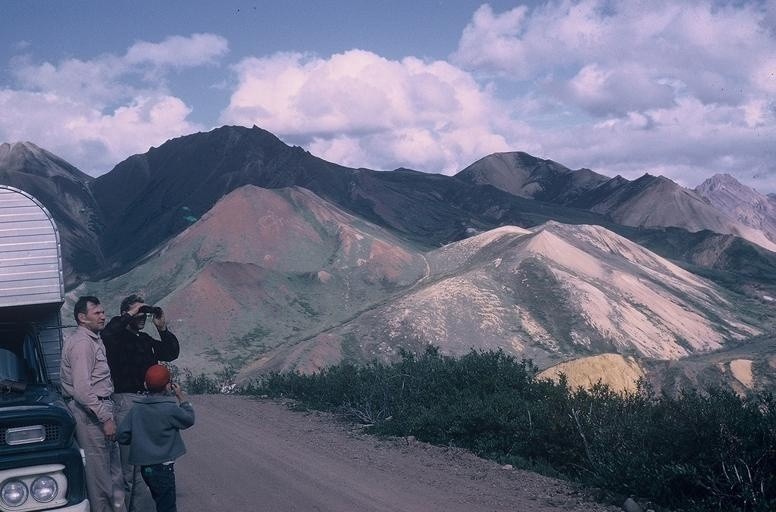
66 395 112 402
112 382 146 397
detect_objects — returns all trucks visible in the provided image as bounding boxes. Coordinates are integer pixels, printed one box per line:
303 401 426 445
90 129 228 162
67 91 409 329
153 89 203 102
0 185 94 512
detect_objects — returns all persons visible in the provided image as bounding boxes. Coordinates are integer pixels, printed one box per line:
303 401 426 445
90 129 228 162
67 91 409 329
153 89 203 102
59 295 128 511
116 364 194 511
100 294 179 511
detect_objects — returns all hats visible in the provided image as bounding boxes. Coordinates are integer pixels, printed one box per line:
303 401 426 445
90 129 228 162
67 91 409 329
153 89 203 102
144 364 170 391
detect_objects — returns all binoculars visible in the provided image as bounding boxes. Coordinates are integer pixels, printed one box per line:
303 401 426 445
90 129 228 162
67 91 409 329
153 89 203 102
138 305 162 315
